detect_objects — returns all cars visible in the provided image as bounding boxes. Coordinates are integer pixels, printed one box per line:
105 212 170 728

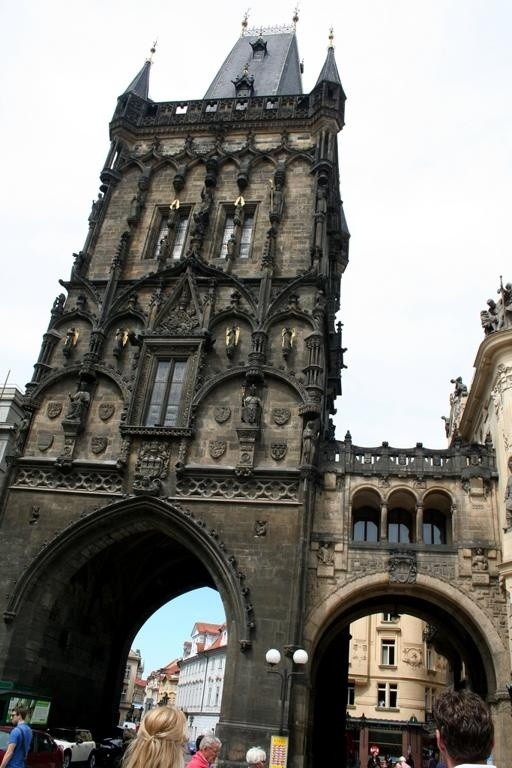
93 725 124 762
0 725 64 768
186 741 196 754
45 726 97 768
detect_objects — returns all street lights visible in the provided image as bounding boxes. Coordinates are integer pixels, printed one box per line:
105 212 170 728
264 648 309 768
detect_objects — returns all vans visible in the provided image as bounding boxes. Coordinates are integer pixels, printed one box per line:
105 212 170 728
117 721 137 732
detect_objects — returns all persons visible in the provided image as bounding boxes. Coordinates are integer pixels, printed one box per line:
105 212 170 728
428 688 497 768
364 742 417 767
244 744 271 767
438 267 512 432
64 381 92 419
0 704 34 767
241 383 263 423
185 734 224 768
121 706 188 768
